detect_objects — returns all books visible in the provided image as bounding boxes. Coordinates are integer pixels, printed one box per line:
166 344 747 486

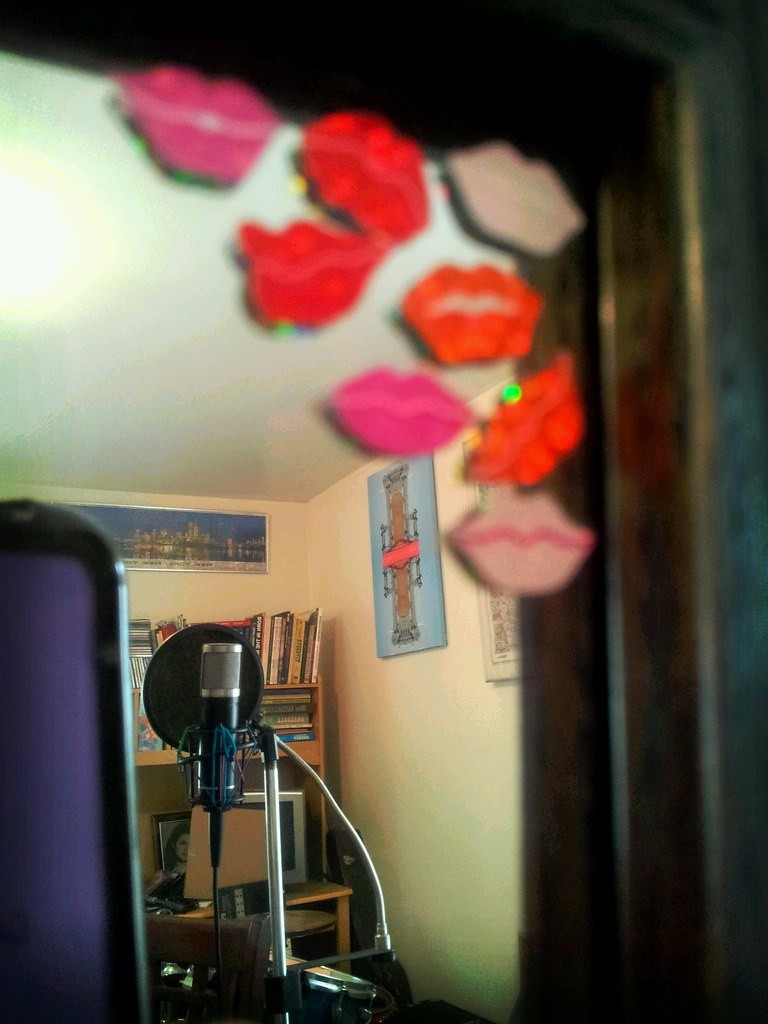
128 607 324 752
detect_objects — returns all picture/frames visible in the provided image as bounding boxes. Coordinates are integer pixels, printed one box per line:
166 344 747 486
50 500 270 576
150 812 191 872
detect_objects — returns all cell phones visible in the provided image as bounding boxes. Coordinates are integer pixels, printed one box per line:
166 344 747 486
0 496 152 1023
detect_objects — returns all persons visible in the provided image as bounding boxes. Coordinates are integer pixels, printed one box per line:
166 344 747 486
166 822 191 874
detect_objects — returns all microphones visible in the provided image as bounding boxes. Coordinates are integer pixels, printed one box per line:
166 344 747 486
199 641 242 868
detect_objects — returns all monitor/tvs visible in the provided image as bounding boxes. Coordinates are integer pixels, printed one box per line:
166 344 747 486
237 791 307 885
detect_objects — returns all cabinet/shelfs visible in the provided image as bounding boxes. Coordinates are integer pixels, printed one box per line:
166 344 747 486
131 676 354 977
